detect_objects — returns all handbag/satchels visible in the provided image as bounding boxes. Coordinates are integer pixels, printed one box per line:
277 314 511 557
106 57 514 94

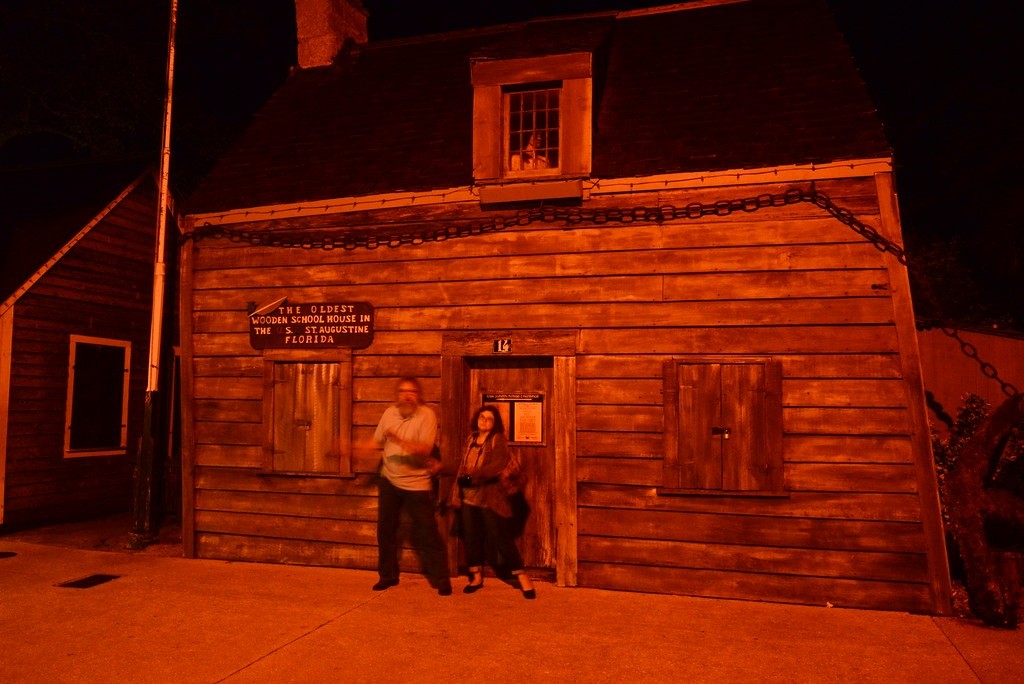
492 435 528 496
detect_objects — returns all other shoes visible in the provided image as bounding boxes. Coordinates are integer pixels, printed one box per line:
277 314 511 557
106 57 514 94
462 581 484 593
373 577 399 590
438 583 451 595
523 586 538 600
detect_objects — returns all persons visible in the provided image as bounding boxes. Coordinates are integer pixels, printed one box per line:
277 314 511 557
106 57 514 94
372 377 453 596
425 407 536 600
